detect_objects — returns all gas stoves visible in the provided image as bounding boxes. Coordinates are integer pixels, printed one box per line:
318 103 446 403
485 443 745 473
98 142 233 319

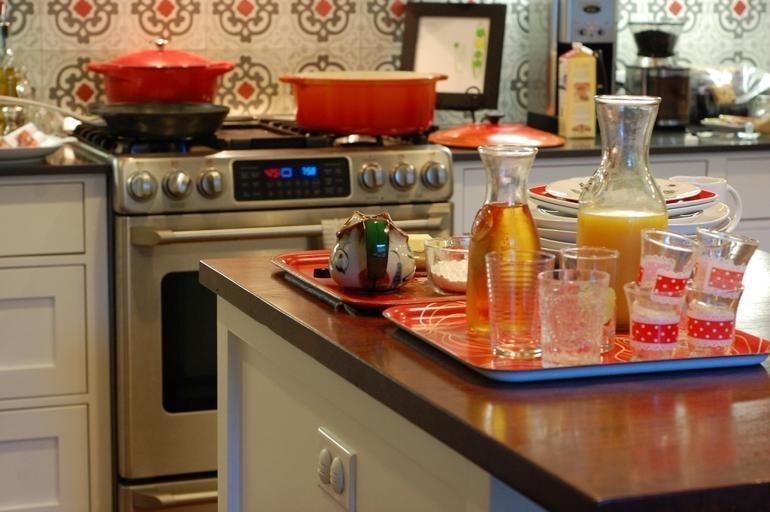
69 117 454 215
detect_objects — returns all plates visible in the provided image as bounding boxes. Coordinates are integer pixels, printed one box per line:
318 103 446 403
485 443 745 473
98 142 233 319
528 177 732 263
0 122 67 164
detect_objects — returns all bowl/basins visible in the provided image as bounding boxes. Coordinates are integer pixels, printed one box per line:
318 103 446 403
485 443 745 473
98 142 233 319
424 238 470 295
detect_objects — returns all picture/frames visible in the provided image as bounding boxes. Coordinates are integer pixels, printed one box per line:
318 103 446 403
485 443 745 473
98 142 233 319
400 2 508 112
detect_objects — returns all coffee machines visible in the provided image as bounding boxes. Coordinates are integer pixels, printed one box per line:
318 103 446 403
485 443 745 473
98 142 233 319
528 0 618 135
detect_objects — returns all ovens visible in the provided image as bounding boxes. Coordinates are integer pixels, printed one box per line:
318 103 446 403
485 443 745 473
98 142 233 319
110 202 453 512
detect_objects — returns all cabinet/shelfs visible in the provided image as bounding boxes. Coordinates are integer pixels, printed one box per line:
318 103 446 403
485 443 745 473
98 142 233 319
0 173 113 511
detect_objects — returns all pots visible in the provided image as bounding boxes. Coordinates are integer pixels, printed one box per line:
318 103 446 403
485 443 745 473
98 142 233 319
84 36 236 140
274 70 448 139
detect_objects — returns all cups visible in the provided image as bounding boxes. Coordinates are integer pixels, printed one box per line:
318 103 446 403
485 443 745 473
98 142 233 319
484 175 759 368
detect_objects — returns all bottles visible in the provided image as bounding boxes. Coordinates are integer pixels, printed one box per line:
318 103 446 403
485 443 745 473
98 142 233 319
467 145 540 341
577 95 668 331
558 43 598 140
624 21 693 129
